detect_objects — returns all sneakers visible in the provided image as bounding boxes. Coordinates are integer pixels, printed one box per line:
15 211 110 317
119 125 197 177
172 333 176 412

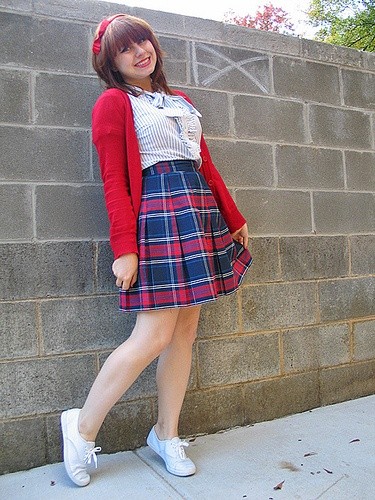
61 408 97 487
145 424 197 477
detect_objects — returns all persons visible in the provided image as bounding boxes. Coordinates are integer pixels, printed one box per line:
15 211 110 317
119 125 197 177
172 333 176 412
59 13 254 488
